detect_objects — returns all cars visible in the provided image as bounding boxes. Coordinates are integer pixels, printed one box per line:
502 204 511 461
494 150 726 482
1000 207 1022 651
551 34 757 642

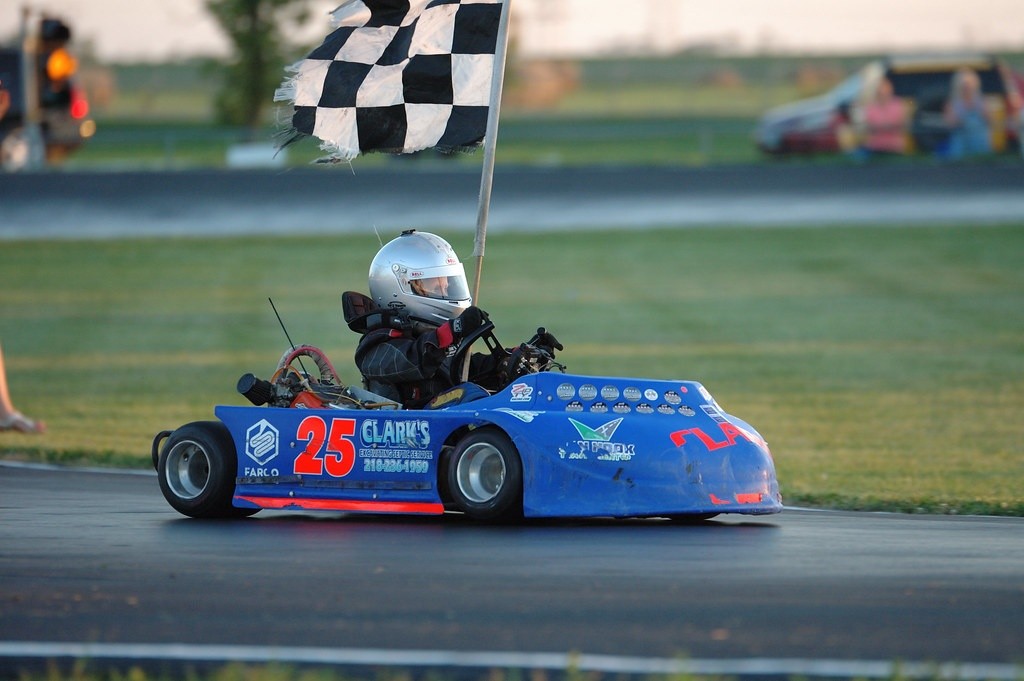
755 53 1024 153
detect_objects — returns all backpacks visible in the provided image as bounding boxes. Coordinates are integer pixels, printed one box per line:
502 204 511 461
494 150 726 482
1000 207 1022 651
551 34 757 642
947 99 993 164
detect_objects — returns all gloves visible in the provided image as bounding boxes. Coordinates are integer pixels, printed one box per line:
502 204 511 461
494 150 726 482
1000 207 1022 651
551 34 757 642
448 305 491 345
527 332 563 359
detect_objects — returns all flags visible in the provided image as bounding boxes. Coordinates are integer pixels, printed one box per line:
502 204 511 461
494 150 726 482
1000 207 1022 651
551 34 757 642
274 0 504 165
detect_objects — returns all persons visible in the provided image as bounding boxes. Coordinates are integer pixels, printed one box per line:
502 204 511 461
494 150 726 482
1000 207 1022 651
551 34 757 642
0 347 46 435
862 78 905 155
341 229 563 410
36 16 80 162
942 69 993 158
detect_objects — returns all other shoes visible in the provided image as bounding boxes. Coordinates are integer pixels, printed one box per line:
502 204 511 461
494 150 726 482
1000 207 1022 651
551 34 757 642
0 413 46 435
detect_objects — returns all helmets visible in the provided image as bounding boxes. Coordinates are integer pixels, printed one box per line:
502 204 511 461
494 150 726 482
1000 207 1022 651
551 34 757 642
369 229 472 326
